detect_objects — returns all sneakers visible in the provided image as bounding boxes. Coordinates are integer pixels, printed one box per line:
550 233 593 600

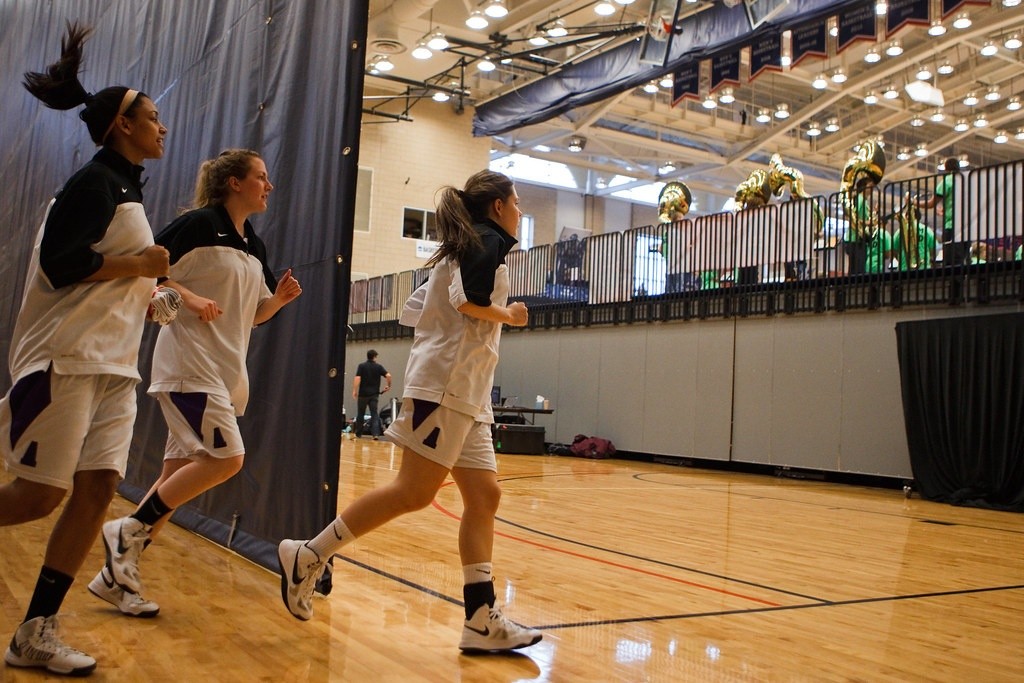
101 514 153 594
87 563 160 617
458 650 540 683
458 604 543 650
278 538 332 620
4 615 96 675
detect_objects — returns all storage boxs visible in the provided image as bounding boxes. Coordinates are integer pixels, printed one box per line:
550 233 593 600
491 423 546 456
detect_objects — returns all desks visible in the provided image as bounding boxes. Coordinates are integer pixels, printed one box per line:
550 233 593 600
493 406 554 424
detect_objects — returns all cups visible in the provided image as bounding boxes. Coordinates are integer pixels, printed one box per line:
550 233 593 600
543 400 549 410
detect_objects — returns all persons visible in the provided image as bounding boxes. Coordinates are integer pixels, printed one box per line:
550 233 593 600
659 158 1022 290
277 170 544 653
88 149 302 618
353 350 391 440
0 18 170 676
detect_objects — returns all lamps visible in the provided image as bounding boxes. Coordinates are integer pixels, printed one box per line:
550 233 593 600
368 0 1024 189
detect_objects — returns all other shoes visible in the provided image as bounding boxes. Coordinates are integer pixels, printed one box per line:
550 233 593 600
372 436 379 439
